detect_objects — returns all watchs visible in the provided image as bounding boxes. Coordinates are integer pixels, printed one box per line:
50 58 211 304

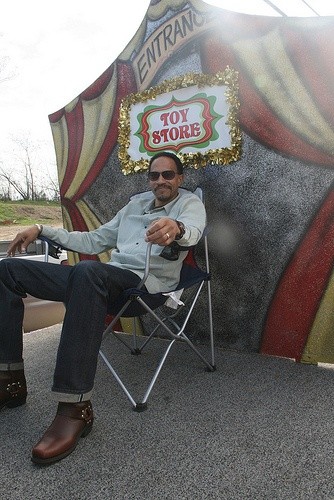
174 219 185 240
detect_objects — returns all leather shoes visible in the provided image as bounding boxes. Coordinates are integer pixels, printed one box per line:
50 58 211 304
0 371 27 409
31 401 94 462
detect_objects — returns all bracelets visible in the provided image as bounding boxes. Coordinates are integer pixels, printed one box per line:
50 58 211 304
35 223 42 232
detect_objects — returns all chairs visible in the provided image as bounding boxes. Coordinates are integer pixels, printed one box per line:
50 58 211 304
39 185 218 414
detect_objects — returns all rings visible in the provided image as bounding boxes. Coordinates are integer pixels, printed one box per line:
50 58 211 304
166 233 170 239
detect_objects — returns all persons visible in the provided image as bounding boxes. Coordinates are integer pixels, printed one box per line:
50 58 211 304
0 152 209 464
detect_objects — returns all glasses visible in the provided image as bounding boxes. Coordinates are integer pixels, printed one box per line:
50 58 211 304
148 170 181 180
